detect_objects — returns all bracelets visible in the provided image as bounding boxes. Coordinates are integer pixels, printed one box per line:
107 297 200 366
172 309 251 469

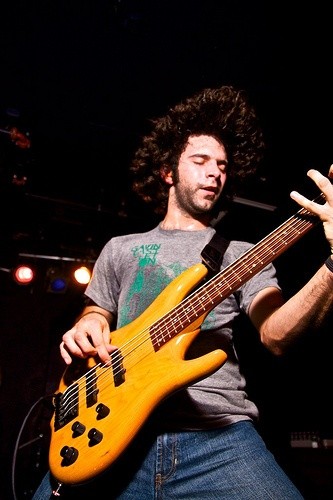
325 255 333 273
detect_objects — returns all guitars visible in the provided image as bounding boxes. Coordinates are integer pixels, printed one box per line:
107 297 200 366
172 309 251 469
48 184 333 489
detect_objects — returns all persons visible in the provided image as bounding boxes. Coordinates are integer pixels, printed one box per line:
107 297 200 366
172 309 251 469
31 87 333 500
